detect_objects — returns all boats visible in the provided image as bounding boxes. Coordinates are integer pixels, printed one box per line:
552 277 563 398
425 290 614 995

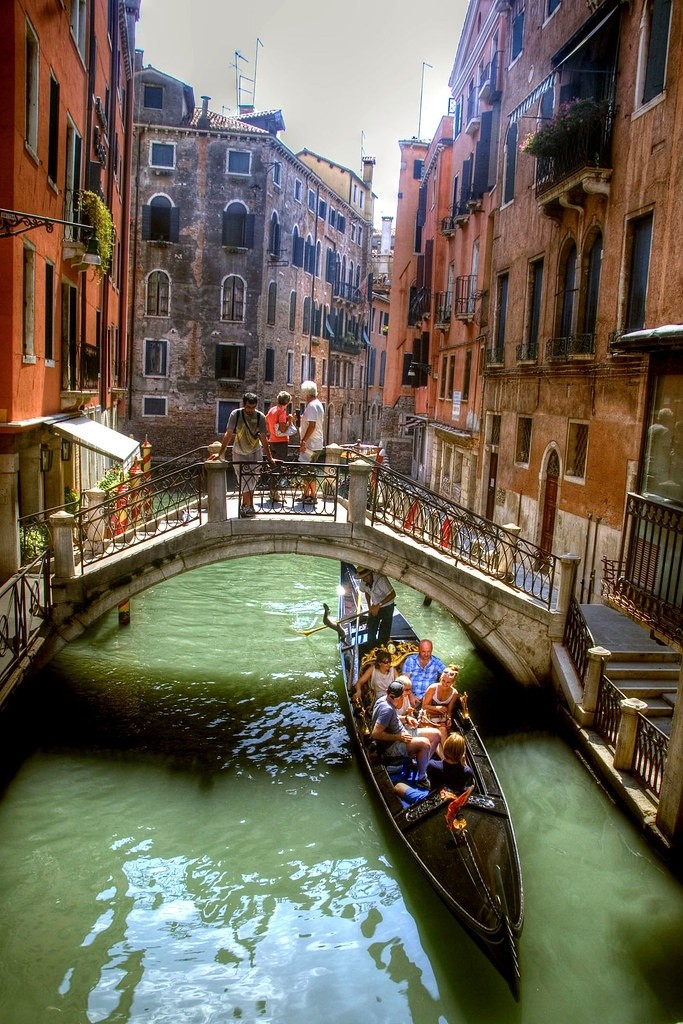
320 553 527 1006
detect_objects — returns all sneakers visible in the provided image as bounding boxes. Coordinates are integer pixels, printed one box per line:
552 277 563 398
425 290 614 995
269 493 285 501
240 504 255 517
304 496 318 504
295 494 309 502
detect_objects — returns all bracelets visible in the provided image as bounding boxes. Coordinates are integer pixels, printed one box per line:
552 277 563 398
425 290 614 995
378 602 382 606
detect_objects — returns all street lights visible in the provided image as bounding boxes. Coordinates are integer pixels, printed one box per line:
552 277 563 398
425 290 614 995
0 207 103 265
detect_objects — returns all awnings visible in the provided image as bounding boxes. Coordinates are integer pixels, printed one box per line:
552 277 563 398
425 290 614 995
52 417 142 471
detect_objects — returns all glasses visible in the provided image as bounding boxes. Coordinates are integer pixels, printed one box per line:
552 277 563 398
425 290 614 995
381 661 391 664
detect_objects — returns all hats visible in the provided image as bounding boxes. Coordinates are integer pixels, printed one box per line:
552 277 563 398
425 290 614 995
354 566 372 578
387 681 405 700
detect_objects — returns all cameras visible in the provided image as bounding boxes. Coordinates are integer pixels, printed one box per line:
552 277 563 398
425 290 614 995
253 431 261 441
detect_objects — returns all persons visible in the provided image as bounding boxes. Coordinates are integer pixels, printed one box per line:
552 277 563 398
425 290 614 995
296 380 325 503
392 731 475 806
394 675 415 722
354 565 396 654
417 664 462 762
352 649 399 703
206 393 276 517
370 680 442 789
354 438 361 452
402 640 448 706
265 390 295 500
